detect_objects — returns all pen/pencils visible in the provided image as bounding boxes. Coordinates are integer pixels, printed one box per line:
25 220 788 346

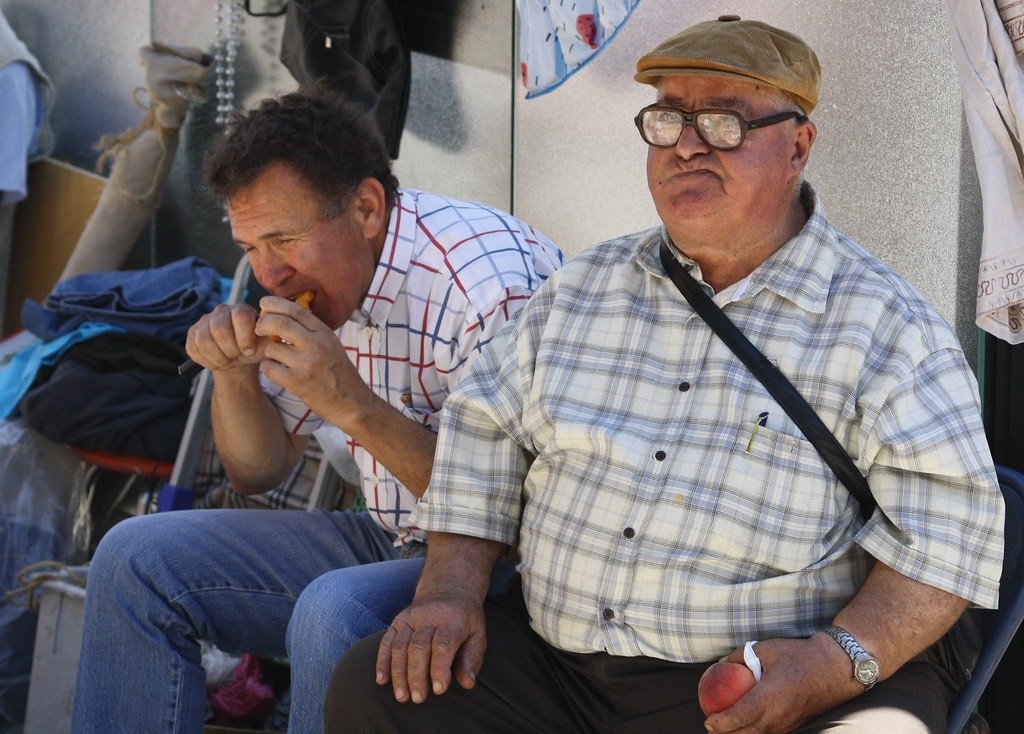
746 411 769 453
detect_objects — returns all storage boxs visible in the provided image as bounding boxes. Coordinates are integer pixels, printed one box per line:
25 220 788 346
21 566 85 734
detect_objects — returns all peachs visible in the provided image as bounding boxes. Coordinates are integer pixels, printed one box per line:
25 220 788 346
697 661 756 718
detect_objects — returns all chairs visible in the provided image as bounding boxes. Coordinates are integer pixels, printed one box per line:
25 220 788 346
951 464 1024 734
70 446 174 539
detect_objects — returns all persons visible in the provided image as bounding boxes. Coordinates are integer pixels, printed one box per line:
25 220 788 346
0 10 59 206
70 87 570 734
323 14 1007 734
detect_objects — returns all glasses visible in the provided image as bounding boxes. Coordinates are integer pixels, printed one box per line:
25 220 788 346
635 102 802 151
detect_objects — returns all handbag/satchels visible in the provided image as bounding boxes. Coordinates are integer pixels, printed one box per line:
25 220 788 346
925 606 985 692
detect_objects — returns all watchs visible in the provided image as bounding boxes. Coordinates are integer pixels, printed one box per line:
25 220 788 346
821 625 880 693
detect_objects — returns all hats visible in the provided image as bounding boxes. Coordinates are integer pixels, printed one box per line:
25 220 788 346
634 15 821 115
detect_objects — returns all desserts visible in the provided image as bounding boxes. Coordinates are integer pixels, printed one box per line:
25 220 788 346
259 290 313 341
